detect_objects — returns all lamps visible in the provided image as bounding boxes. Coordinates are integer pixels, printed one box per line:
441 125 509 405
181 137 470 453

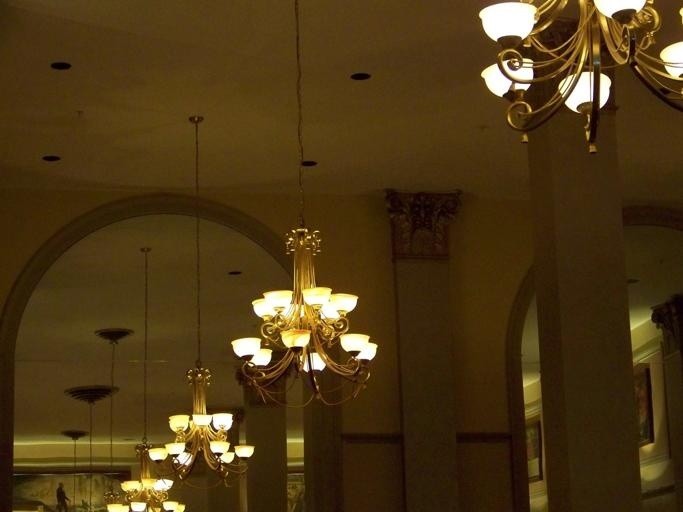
148 114 255 490
229 0 379 412
472 0 683 156
103 246 185 512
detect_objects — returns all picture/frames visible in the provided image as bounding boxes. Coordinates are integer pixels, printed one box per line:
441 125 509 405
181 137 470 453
11 469 131 512
286 471 305 512
524 420 543 483
631 367 654 448
